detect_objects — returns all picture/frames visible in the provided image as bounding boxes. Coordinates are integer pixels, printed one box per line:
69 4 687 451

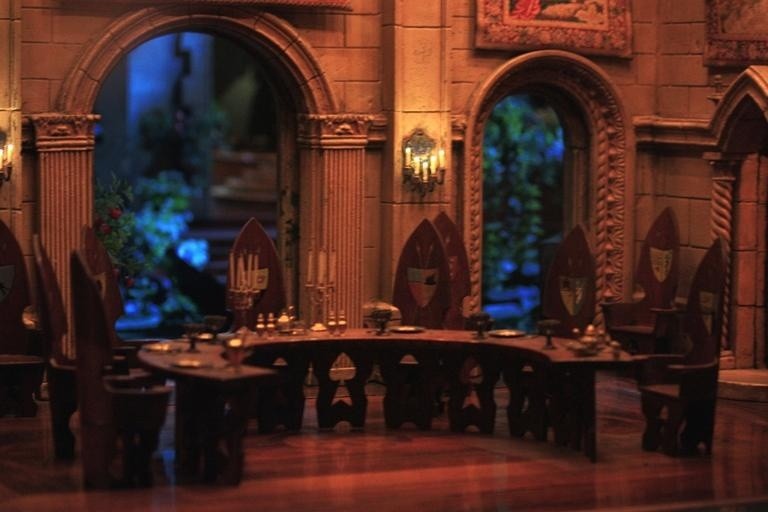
702 0 768 66
475 1 634 61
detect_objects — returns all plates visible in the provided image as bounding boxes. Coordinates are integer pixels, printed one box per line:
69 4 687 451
486 328 528 338
174 361 207 368
389 325 425 333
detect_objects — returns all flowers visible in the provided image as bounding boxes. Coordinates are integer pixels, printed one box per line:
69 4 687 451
92 167 157 289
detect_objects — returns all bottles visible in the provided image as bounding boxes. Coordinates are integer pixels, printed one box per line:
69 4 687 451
328 308 347 337
255 312 276 338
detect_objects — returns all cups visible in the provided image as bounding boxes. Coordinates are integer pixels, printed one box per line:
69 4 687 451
224 337 255 373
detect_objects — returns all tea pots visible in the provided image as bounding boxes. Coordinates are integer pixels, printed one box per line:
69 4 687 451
574 326 607 355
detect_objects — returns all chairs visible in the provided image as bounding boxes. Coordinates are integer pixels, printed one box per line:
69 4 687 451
30 206 728 489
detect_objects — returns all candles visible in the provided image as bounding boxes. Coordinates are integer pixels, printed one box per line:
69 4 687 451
0 144 13 170
405 146 445 181
